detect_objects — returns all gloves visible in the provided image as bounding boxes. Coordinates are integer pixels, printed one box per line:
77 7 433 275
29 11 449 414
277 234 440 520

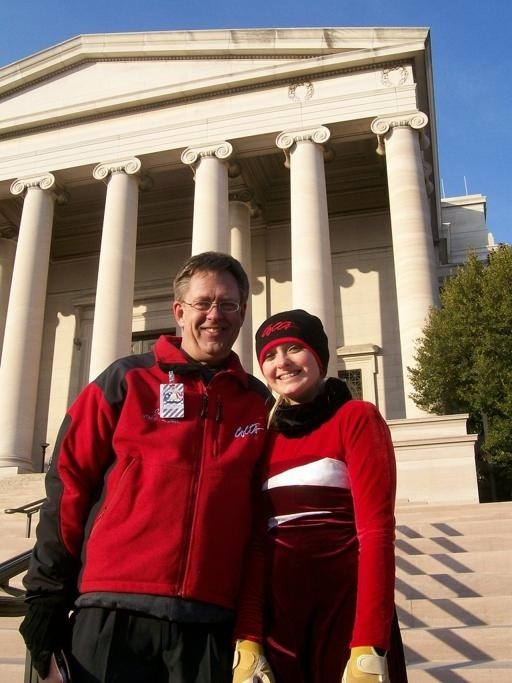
20 607 62 678
342 647 390 683
232 639 275 683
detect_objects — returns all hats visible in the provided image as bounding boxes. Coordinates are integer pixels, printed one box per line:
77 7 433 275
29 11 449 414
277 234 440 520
255 309 329 376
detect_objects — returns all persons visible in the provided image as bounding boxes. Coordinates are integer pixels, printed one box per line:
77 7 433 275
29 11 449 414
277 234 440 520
227 306 410 682
16 247 276 681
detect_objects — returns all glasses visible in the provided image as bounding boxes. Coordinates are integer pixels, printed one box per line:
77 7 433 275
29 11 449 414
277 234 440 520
183 300 245 313
